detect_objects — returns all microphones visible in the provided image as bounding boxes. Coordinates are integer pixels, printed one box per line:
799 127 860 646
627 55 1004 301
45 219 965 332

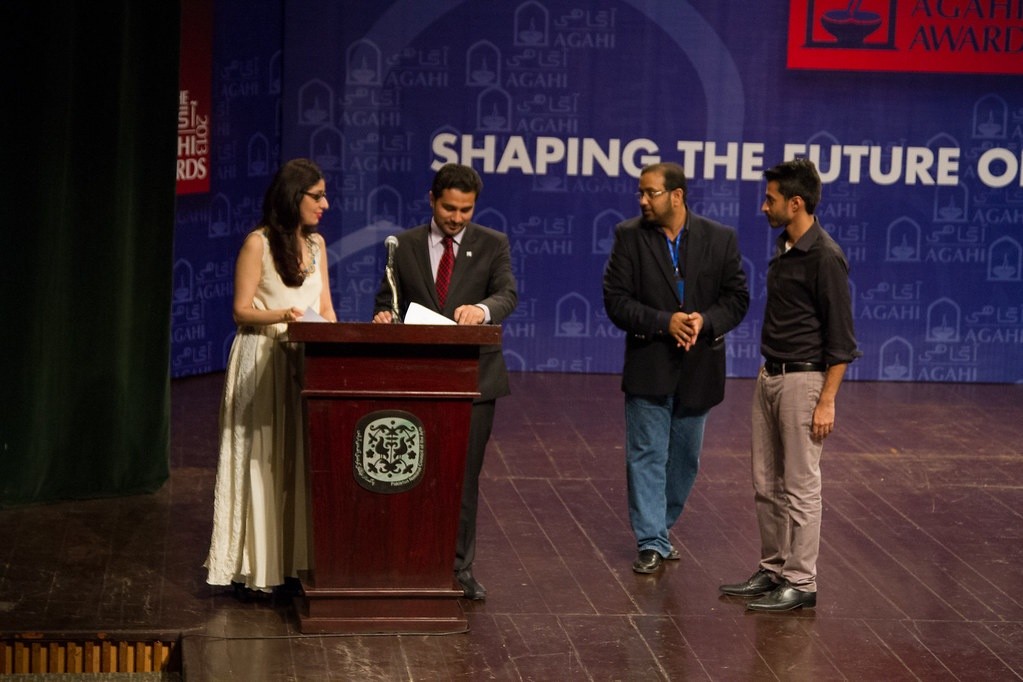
385 234 399 270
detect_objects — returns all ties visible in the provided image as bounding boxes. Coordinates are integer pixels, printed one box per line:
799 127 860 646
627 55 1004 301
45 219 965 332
435 237 455 310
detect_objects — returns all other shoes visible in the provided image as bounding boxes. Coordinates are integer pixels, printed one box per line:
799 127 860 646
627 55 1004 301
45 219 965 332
233 583 259 602
284 576 306 597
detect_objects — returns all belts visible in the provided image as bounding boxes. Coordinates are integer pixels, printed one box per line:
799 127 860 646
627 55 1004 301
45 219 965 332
764 358 827 377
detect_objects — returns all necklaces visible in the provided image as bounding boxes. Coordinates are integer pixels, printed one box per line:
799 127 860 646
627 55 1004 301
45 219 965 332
304 236 316 276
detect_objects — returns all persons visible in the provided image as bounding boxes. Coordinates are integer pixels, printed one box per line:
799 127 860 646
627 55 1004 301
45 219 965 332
372 163 518 601
200 157 338 593
603 161 750 574
718 158 864 612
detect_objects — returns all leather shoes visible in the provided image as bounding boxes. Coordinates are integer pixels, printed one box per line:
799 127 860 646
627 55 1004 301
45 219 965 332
632 549 662 573
665 535 681 560
455 568 488 601
747 582 816 613
719 569 784 597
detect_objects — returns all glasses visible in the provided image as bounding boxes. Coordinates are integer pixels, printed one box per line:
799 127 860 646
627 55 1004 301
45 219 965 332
633 189 675 202
300 191 327 200
